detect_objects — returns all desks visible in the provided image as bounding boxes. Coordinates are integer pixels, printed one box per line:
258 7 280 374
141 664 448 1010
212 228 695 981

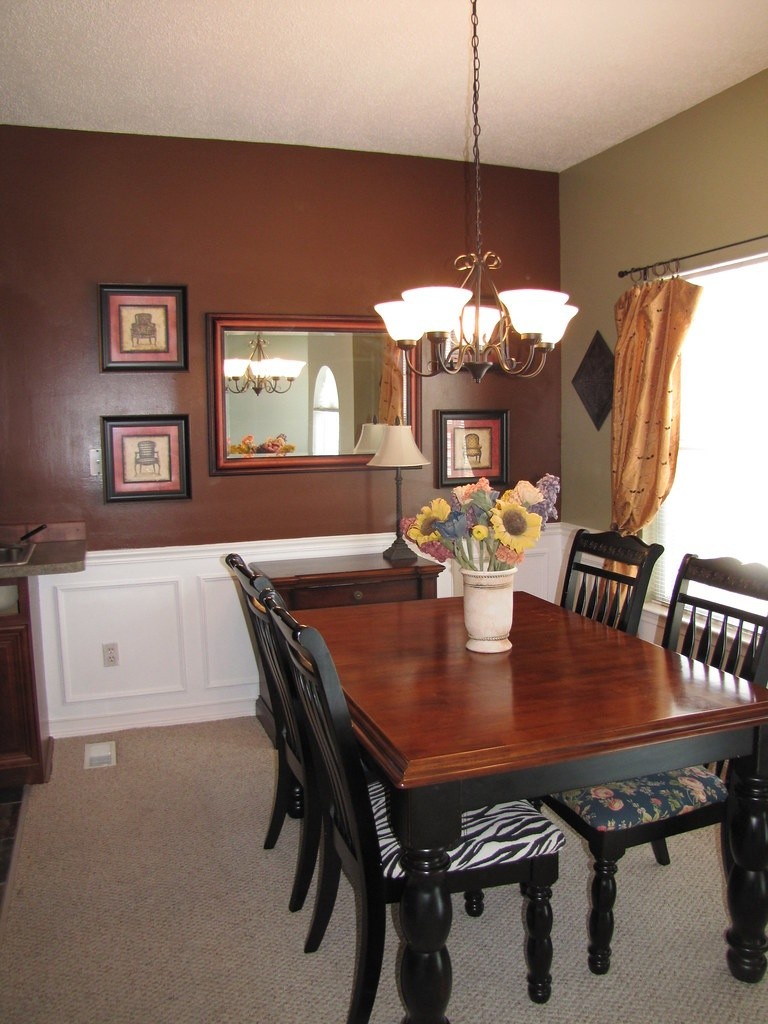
286 591 768 1024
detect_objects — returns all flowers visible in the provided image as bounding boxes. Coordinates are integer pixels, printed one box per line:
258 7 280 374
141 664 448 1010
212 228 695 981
398 472 560 572
228 432 297 456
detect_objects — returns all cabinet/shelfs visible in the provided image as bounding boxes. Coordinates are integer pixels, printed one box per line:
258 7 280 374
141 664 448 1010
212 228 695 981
0 577 54 789
247 552 446 611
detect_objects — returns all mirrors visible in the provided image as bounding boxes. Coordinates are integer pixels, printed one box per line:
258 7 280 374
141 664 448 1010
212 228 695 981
205 312 423 477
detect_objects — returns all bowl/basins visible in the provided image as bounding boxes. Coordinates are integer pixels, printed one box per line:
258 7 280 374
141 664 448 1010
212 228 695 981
0 585 19 611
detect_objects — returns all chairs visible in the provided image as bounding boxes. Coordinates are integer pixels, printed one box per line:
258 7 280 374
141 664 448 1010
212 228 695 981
529 553 768 976
257 587 567 1024
560 528 665 636
225 553 378 913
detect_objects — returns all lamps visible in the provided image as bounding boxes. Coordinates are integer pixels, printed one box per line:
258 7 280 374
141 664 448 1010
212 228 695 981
366 415 432 563
353 414 388 454
223 331 306 396
372 0 580 387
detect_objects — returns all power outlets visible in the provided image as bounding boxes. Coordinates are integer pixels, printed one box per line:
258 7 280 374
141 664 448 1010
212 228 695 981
102 642 120 667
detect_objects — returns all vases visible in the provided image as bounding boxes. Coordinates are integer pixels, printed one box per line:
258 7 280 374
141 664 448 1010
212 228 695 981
458 563 519 654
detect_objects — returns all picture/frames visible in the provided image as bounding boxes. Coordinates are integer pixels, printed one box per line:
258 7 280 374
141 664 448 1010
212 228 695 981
432 407 511 489
100 413 193 505
97 282 191 374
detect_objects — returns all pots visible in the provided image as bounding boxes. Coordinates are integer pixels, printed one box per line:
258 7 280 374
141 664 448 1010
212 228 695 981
0 523 47 567
